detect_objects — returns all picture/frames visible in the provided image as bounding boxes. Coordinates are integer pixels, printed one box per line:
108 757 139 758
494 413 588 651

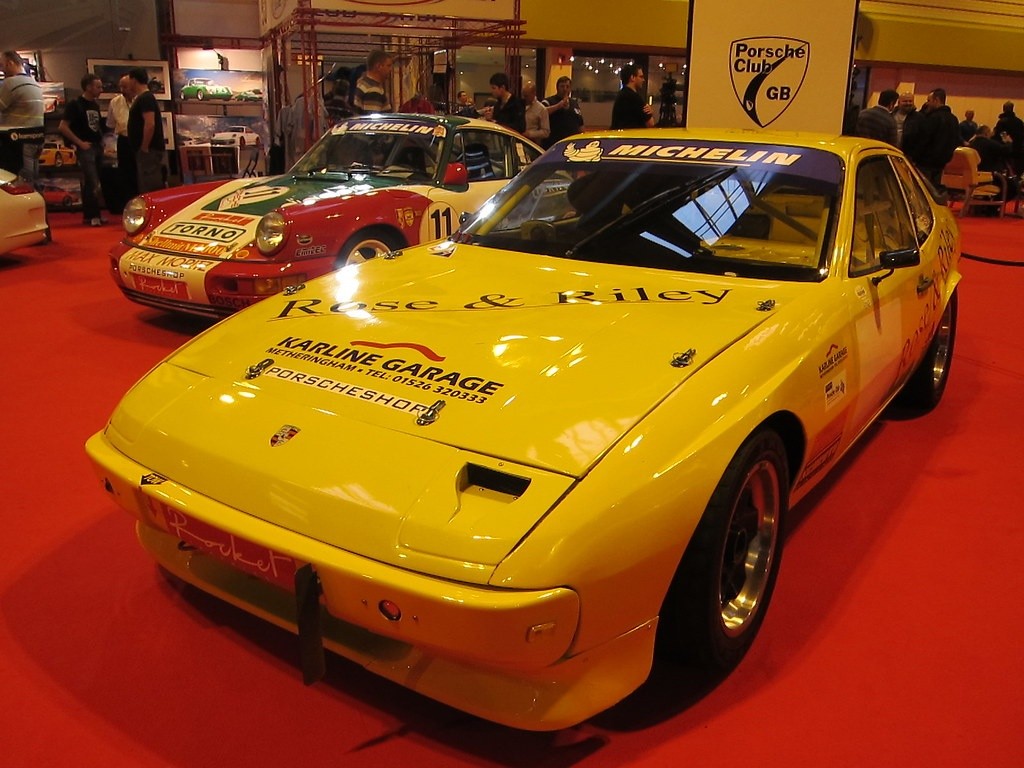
100 110 176 151
86 57 170 101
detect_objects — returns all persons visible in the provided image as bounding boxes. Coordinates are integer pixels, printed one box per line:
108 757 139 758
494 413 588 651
455 90 481 143
56 73 103 229
0 50 54 246
484 73 526 144
845 87 1024 217
107 66 170 194
541 76 584 151
353 49 393 165
611 62 657 129
520 83 552 162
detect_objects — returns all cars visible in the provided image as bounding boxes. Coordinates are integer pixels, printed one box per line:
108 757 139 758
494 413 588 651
178 78 233 102
1 170 52 251
37 140 79 174
34 175 99 209
209 126 261 147
235 88 264 102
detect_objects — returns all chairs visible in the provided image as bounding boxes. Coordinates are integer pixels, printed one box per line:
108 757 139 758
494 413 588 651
940 146 1006 219
458 142 496 180
396 145 428 173
364 142 387 166
565 174 623 241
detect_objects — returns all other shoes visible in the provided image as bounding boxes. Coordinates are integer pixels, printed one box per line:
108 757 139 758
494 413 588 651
91 218 101 226
84 216 107 223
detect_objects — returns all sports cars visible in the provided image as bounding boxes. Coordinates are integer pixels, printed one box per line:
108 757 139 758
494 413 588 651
107 113 588 322
80 126 963 732
